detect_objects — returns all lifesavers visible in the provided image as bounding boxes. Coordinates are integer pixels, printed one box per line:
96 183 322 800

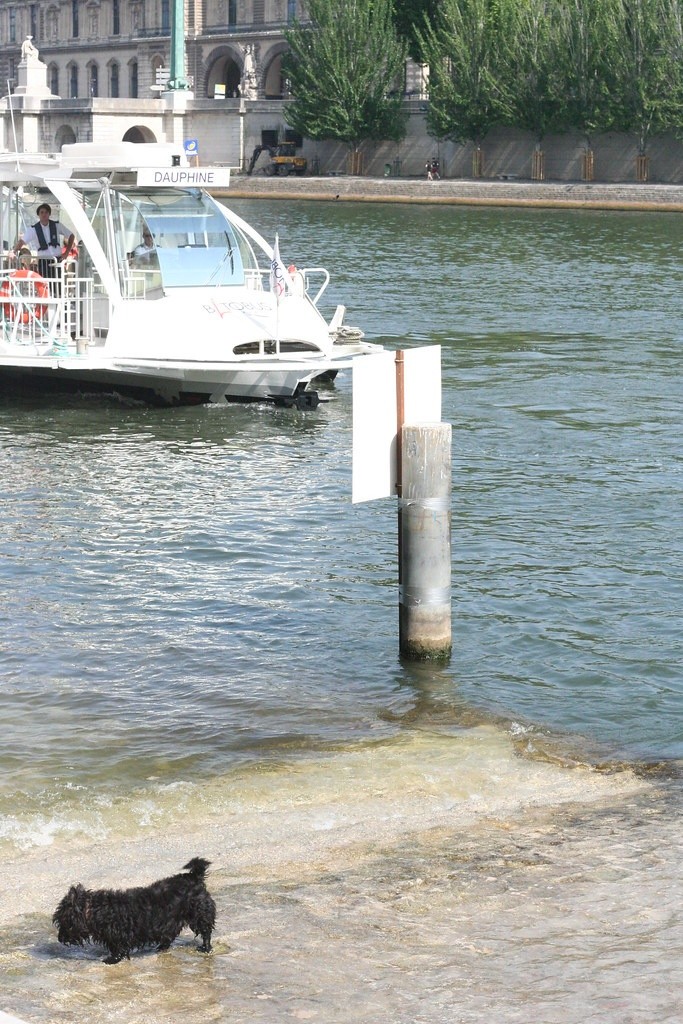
0 270 49 323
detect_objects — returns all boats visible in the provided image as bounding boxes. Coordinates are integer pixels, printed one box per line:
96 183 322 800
0 33 389 411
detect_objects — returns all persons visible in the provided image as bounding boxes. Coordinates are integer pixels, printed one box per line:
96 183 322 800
431 157 440 179
425 160 433 181
14 239 27 256
22 36 38 60
8 203 74 296
135 233 161 257
19 248 31 264
61 237 78 257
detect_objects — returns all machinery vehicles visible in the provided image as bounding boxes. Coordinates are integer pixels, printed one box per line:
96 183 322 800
243 141 308 176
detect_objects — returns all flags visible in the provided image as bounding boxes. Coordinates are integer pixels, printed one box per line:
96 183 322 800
269 237 286 309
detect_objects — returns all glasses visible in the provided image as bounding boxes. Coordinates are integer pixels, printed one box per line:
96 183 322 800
145 233 153 237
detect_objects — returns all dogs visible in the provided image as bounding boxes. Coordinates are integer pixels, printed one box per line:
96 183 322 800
53 858 218 965
